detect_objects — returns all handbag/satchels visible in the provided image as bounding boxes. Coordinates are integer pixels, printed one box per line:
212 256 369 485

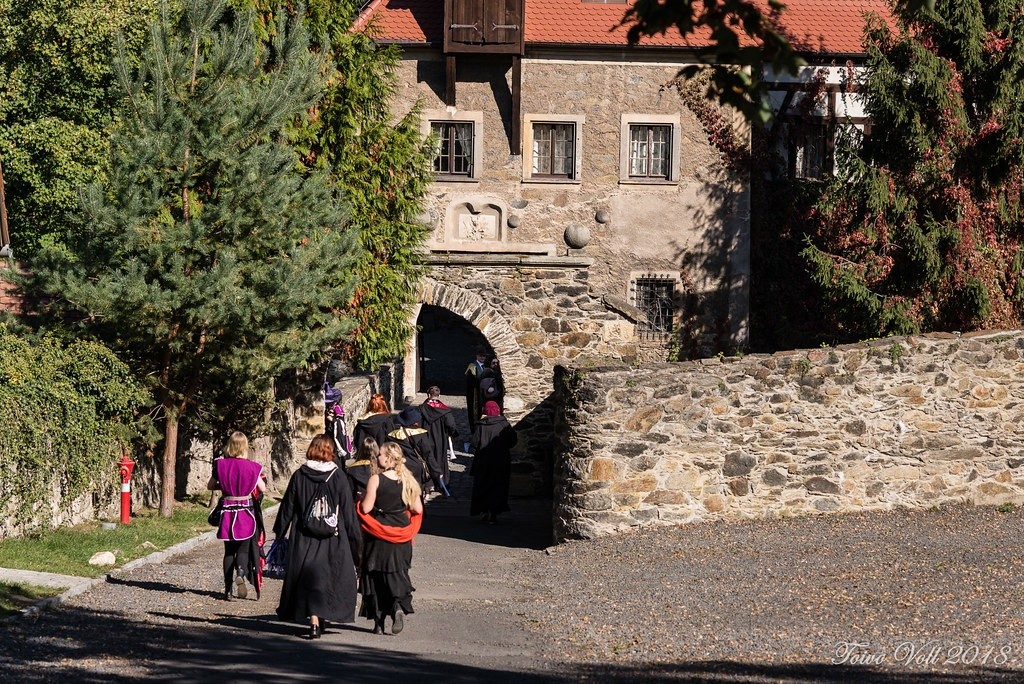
418 456 431 483
268 537 289 578
208 506 221 526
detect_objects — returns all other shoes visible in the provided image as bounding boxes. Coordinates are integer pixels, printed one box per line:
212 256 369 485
480 512 489 521
372 619 384 635
488 517 496 525
310 619 325 638
391 603 404 634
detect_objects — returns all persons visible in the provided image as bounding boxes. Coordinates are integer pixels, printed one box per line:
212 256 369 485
207 430 266 602
466 347 505 433
274 435 423 639
324 385 518 525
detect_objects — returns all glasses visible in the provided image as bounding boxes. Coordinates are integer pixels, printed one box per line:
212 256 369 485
480 355 485 357
493 362 499 364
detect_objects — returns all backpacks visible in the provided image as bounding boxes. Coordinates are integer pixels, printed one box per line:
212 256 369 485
300 467 339 539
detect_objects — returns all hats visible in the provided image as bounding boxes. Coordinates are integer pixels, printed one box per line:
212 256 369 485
471 345 490 356
397 407 422 426
484 401 500 416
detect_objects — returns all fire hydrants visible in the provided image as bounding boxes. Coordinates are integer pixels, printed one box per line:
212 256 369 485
116 456 136 525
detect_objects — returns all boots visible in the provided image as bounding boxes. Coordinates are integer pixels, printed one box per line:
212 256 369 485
235 569 247 599
225 578 233 600
